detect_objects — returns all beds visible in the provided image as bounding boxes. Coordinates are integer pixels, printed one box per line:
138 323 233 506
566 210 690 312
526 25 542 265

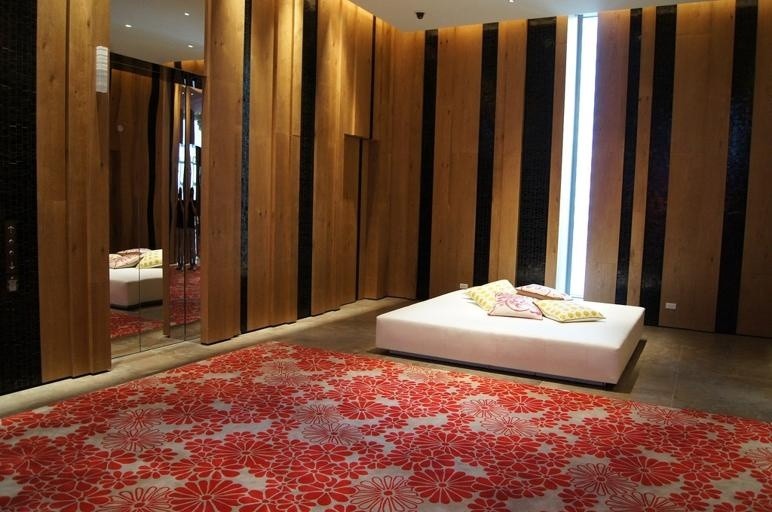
110 268 169 309
375 288 645 389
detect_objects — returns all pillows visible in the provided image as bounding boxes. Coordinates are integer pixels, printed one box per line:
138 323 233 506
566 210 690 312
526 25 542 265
467 279 605 322
110 247 164 268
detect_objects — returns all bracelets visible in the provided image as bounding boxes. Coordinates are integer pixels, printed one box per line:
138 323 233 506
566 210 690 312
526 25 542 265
191 206 194 208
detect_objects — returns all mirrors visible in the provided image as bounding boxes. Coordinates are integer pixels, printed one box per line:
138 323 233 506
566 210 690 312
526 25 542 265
110 0 202 354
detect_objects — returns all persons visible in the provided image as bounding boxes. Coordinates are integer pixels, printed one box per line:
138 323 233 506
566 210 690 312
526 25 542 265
187 187 198 271
175 188 184 272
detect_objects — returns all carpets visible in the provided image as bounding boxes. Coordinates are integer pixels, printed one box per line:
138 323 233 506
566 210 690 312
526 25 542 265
0 339 772 512
